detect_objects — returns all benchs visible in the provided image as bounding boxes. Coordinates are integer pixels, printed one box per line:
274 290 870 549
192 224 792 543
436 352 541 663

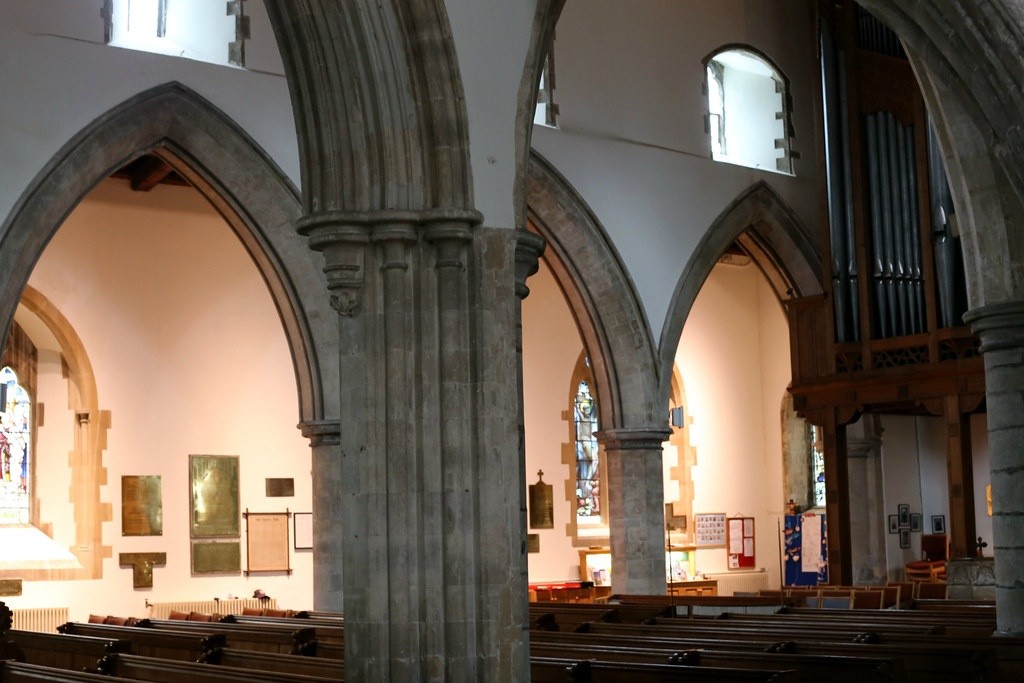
528 594 1024 683
0 612 346 683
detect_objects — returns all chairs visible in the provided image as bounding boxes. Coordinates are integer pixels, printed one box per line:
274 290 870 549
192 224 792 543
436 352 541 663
88 606 296 626
780 535 951 608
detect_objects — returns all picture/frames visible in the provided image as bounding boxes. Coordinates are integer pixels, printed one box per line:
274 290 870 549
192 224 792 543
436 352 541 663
122 476 162 536
189 455 241 538
931 516 944 533
910 513 921 531
293 511 313 550
900 528 911 548
191 542 241 577
898 504 910 526
890 515 898 533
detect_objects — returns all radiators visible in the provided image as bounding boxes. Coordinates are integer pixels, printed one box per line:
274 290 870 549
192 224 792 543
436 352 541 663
218 597 277 614
706 572 769 597
150 598 217 620
10 607 69 632
